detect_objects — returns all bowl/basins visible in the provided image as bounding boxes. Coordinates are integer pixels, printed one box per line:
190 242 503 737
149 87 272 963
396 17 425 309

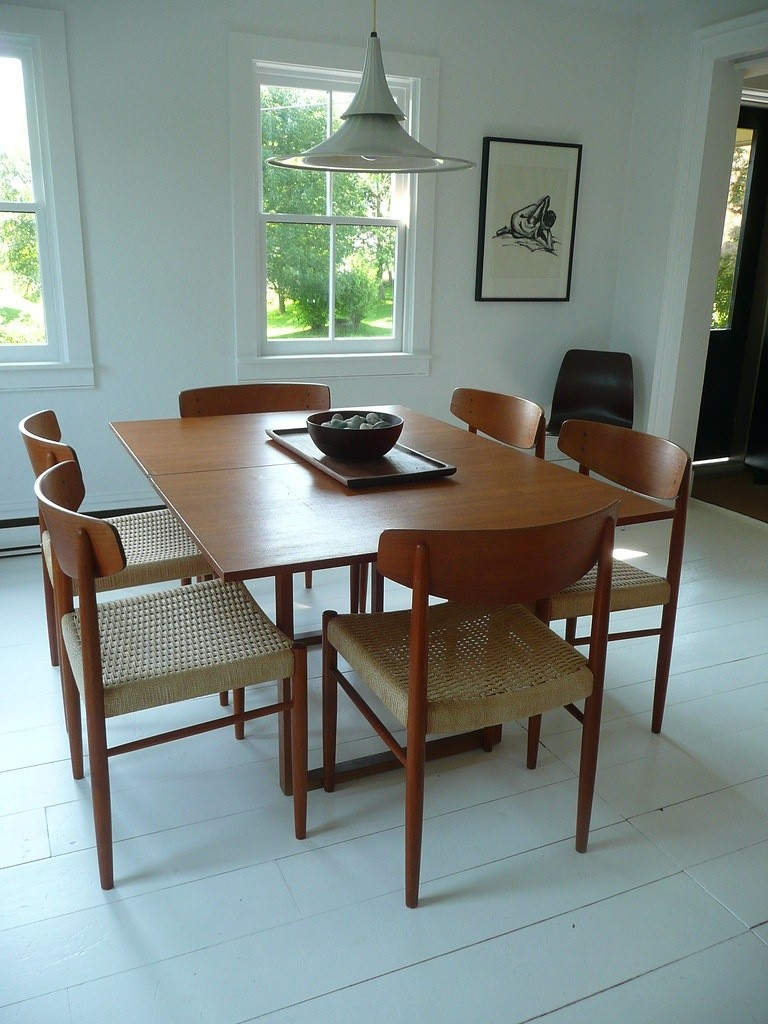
306 411 405 461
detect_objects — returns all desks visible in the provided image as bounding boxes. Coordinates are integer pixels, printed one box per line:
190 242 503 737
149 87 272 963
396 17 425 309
108 404 679 796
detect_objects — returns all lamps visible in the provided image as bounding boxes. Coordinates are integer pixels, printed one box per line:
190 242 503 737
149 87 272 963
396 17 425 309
263 0 478 173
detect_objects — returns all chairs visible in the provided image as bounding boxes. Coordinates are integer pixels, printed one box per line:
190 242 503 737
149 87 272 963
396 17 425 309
544 350 634 437
450 387 691 736
19 408 308 890
176 384 331 588
321 498 622 909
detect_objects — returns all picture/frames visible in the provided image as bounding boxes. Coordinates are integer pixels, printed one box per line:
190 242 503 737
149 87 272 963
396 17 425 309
475 136 583 303
